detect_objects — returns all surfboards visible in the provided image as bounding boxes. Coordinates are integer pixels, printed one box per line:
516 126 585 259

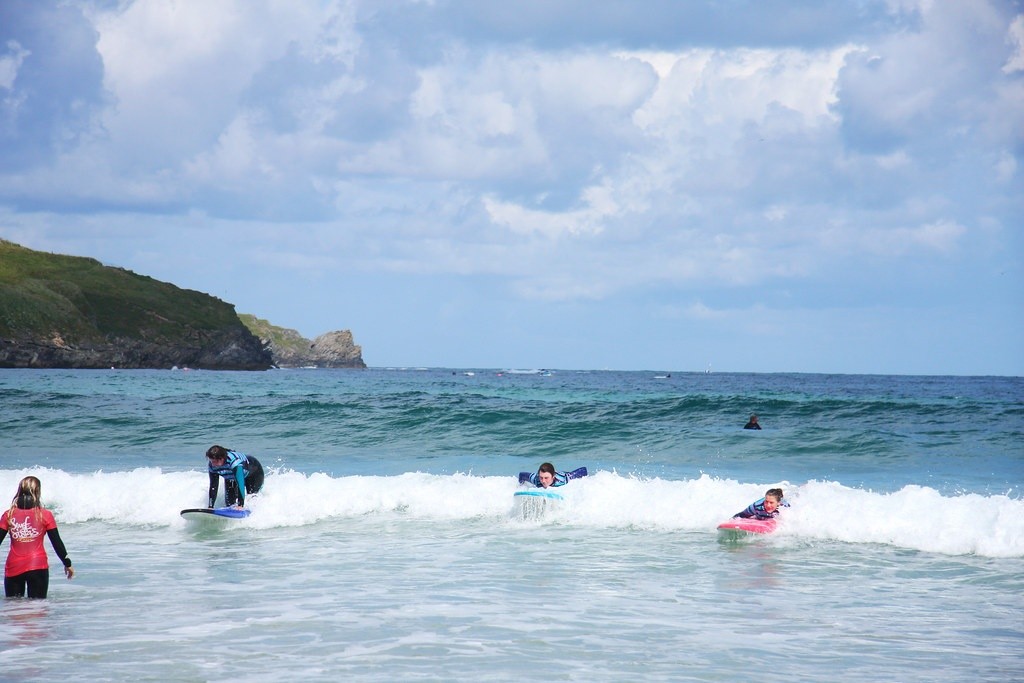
513 491 563 500
180 507 251 519
717 517 777 534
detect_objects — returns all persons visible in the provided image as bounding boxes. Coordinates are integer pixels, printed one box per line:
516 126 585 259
733 488 790 521
519 463 587 488
206 445 264 510
0 476 74 598
744 416 761 430
